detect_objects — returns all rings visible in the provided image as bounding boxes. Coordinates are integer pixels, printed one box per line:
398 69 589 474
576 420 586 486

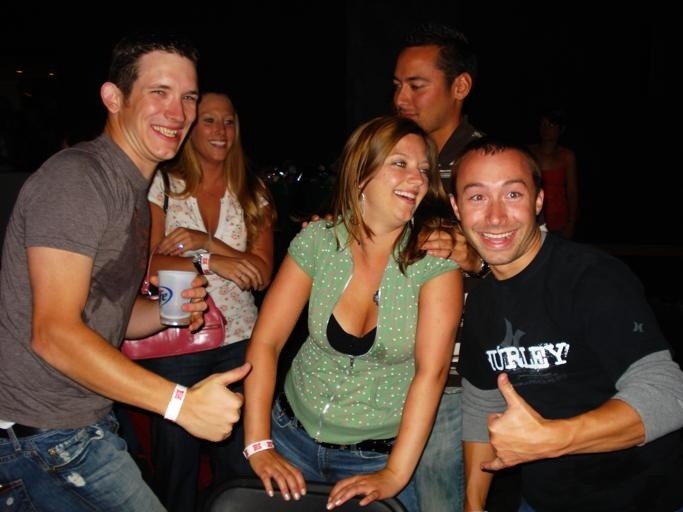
177 242 183 249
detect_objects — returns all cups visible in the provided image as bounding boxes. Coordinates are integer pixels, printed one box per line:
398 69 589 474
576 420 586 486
158 269 197 327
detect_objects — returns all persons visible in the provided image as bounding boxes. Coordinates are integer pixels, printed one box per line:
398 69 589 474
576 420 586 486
1 35 253 511
123 86 275 510
446 134 681 511
219 112 466 511
528 112 579 239
295 28 554 511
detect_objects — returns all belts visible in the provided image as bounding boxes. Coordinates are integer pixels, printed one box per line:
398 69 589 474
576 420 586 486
277 386 397 454
0 423 40 438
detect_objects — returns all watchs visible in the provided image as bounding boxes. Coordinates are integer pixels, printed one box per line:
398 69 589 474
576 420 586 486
467 256 489 279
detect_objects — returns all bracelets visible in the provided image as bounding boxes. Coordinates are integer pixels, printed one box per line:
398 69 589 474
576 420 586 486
242 438 274 459
163 383 186 423
200 253 212 275
191 249 203 275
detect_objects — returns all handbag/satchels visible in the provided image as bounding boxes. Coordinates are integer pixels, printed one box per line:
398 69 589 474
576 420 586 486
118 237 227 360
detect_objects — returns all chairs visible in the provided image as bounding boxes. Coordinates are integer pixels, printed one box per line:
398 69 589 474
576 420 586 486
201 478 409 512
